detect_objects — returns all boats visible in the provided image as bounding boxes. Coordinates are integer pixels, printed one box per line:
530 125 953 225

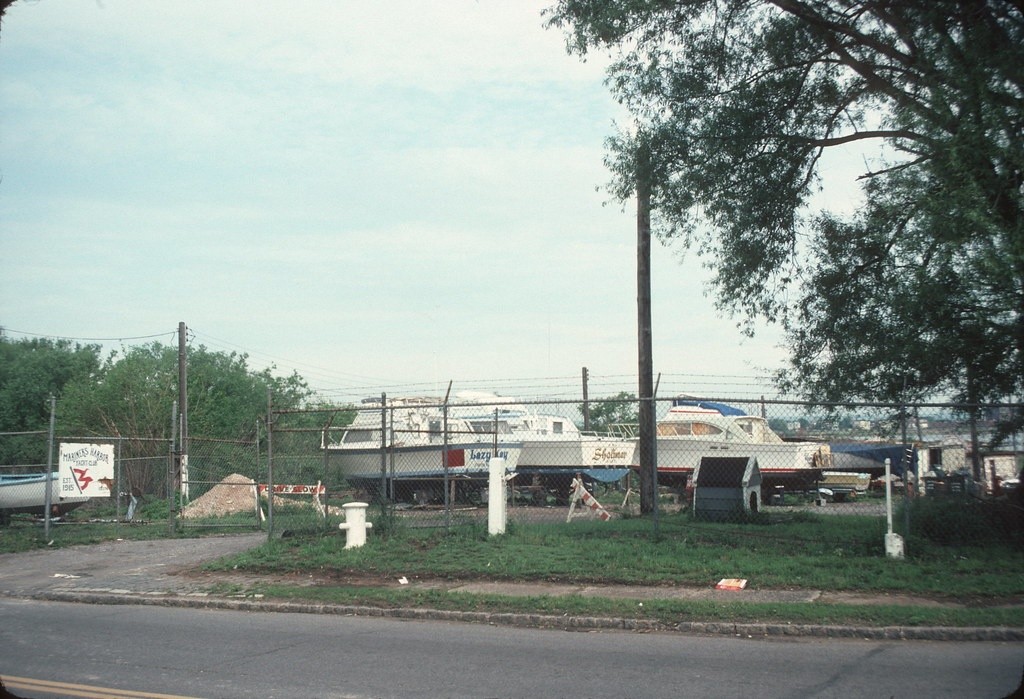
605 403 830 502
0 466 91 527
667 395 919 499
318 388 640 505
